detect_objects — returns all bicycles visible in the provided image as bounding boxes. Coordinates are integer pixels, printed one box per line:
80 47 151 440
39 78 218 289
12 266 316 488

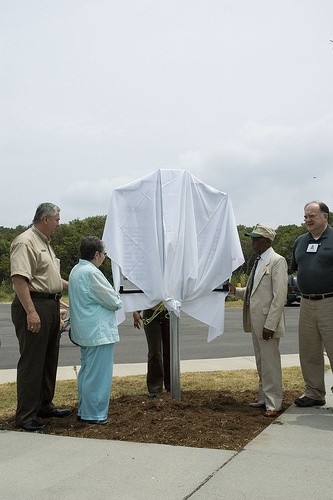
59 318 82 348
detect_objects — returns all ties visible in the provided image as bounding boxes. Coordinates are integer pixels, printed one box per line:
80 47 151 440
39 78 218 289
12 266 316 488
250 256 261 296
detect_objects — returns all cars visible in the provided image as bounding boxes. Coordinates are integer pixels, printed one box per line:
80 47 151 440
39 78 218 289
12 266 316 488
285 274 302 303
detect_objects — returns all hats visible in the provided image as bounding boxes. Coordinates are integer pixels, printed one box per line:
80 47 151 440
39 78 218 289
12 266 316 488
244 223 276 242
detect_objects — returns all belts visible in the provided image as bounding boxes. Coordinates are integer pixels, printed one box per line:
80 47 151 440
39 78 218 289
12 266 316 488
30 291 63 301
301 293 333 300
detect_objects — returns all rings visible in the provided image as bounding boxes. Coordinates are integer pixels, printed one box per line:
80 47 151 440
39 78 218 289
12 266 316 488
134 323 136 324
31 326 34 328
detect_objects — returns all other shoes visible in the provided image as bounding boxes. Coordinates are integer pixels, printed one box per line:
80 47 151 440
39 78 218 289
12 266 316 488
294 396 326 407
147 385 164 398
77 416 108 425
249 402 265 408
264 410 278 416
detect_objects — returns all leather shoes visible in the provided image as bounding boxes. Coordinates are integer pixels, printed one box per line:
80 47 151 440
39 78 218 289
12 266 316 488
15 420 46 432
37 407 71 418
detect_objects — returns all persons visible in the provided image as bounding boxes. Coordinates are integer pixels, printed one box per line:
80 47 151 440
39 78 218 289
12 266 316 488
228 223 288 417
10 202 76 430
69 237 123 426
288 201 333 407
133 301 171 398
